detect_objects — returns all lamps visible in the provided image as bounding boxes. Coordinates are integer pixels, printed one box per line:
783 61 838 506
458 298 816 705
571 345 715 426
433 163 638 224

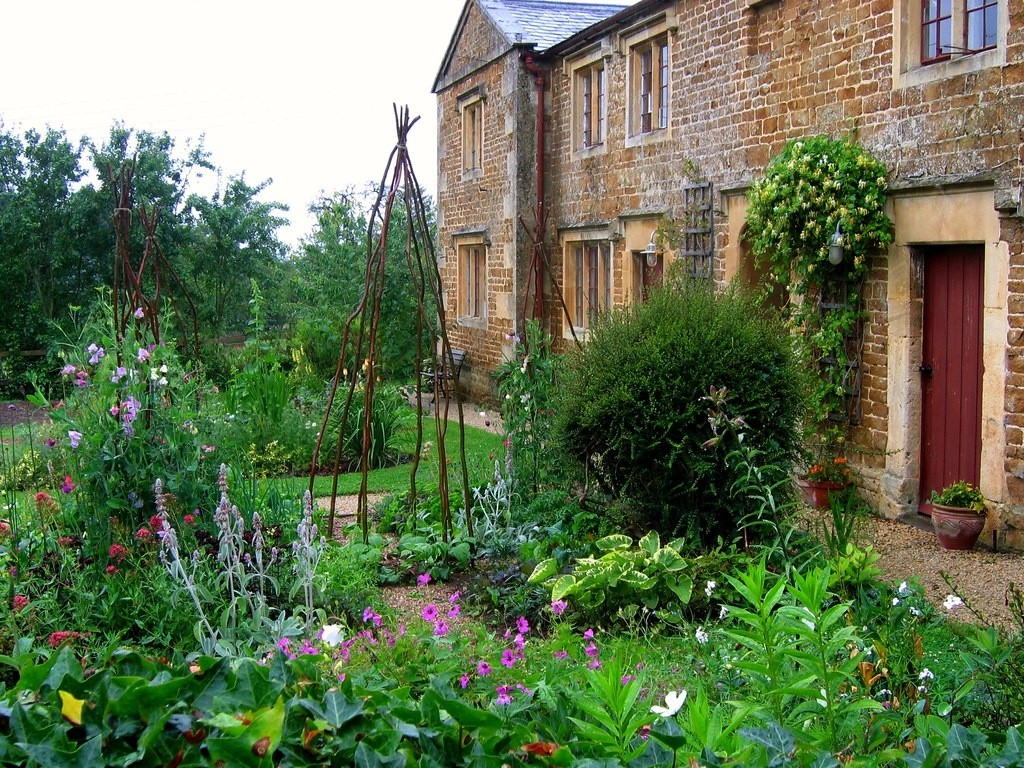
828 220 846 265
639 230 660 267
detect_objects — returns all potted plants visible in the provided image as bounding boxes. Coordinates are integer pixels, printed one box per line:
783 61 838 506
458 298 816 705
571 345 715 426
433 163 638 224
926 480 988 550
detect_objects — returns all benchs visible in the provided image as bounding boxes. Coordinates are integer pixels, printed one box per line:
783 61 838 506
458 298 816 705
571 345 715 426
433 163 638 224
414 349 468 403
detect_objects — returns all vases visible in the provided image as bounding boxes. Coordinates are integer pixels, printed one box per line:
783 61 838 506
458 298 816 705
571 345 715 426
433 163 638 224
809 482 854 511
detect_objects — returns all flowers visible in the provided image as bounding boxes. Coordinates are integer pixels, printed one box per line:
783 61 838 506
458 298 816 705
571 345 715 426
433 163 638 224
805 457 857 483
